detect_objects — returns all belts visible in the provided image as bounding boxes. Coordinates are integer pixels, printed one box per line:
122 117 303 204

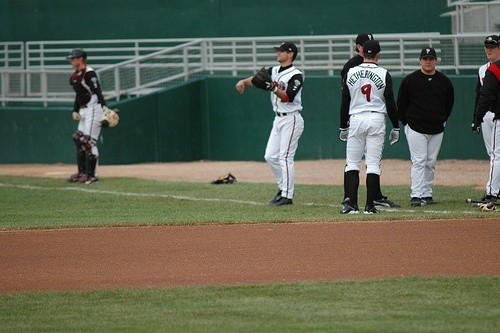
276 112 287 118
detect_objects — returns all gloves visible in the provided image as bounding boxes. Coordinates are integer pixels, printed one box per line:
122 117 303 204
471 119 482 134
338 128 348 142
389 128 401 145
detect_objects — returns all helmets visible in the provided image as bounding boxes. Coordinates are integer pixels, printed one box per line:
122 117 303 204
99 106 120 128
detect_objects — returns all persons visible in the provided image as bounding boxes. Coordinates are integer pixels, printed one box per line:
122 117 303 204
66 50 107 183
236 41 305 206
396 47 454 206
339 32 400 215
472 35 500 211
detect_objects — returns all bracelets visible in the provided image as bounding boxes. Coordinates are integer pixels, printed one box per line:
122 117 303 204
273 87 277 92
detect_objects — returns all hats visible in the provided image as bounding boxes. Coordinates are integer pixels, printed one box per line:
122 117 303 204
273 41 298 56
483 35 500 48
355 32 375 47
419 47 437 58
66 49 88 60
363 40 382 54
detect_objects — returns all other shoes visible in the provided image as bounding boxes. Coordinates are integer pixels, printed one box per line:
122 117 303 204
363 205 380 215
340 198 360 215
372 196 400 209
78 175 97 184
67 173 83 182
410 197 421 207
267 190 283 205
271 196 293 207
471 194 500 208
421 197 437 205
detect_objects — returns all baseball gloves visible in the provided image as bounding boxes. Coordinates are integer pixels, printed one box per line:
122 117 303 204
251 66 278 92
99 107 120 127
